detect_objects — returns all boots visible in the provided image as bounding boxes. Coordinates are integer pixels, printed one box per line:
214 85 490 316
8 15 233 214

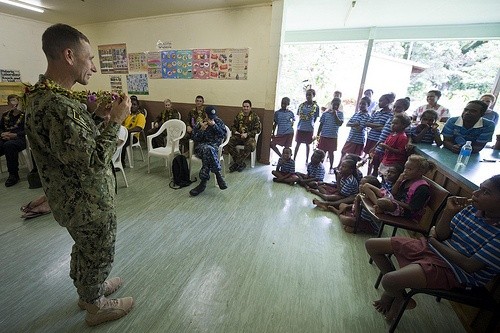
228 149 250 172
78 277 122 310
190 180 207 196
85 295 134 326
214 171 228 190
4 162 20 187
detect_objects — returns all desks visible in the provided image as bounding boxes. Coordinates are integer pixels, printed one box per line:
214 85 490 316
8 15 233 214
414 141 500 329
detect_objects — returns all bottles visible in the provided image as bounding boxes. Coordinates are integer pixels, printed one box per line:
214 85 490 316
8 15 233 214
454 141 472 172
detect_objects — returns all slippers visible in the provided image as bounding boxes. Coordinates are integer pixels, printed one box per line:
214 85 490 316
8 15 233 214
20 201 33 213
21 209 51 218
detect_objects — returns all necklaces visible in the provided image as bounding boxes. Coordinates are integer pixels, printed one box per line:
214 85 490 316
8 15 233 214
191 107 205 126
162 109 173 123
299 101 317 120
239 111 254 134
5 110 23 129
39 74 124 105
123 112 138 128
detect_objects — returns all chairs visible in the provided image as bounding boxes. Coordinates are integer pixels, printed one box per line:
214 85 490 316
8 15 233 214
110 125 129 188
147 119 186 179
141 108 147 150
188 124 232 188
162 112 181 140
18 134 33 172
227 122 263 168
374 253 493 333
353 174 452 265
126 131 145 169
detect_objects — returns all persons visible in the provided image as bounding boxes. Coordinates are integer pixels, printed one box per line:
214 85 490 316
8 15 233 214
24 22 132 325
364 175 500 321
270 89 499 232
0 93 261 218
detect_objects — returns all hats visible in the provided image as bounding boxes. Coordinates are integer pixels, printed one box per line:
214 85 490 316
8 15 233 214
206 106 218 119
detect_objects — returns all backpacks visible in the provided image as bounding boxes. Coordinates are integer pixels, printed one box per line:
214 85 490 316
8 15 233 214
169 155 197 189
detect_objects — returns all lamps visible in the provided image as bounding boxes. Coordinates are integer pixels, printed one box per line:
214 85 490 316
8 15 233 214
0 0 47 14
